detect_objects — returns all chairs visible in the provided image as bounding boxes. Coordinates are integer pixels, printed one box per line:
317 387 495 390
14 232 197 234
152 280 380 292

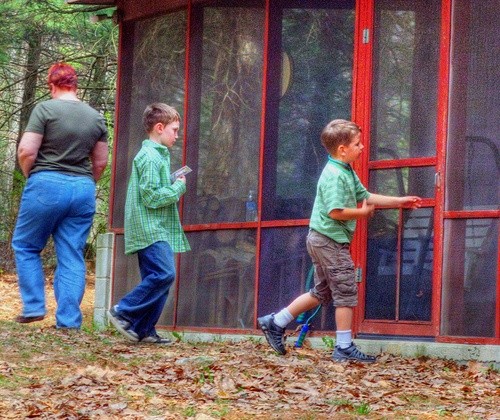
270 191 495 321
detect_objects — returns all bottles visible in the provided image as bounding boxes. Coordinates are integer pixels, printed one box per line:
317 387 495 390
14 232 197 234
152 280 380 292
246 191 257 222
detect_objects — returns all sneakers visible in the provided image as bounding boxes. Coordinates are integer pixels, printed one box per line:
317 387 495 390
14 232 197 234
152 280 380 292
139 332 170 344
15 315 44 323
257 312 287 355
332 342 376 363
106 304 139 342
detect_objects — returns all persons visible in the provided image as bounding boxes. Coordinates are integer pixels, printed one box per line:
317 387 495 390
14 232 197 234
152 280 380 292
11 62 108 329
106 103 193 343
257 119 423 363
